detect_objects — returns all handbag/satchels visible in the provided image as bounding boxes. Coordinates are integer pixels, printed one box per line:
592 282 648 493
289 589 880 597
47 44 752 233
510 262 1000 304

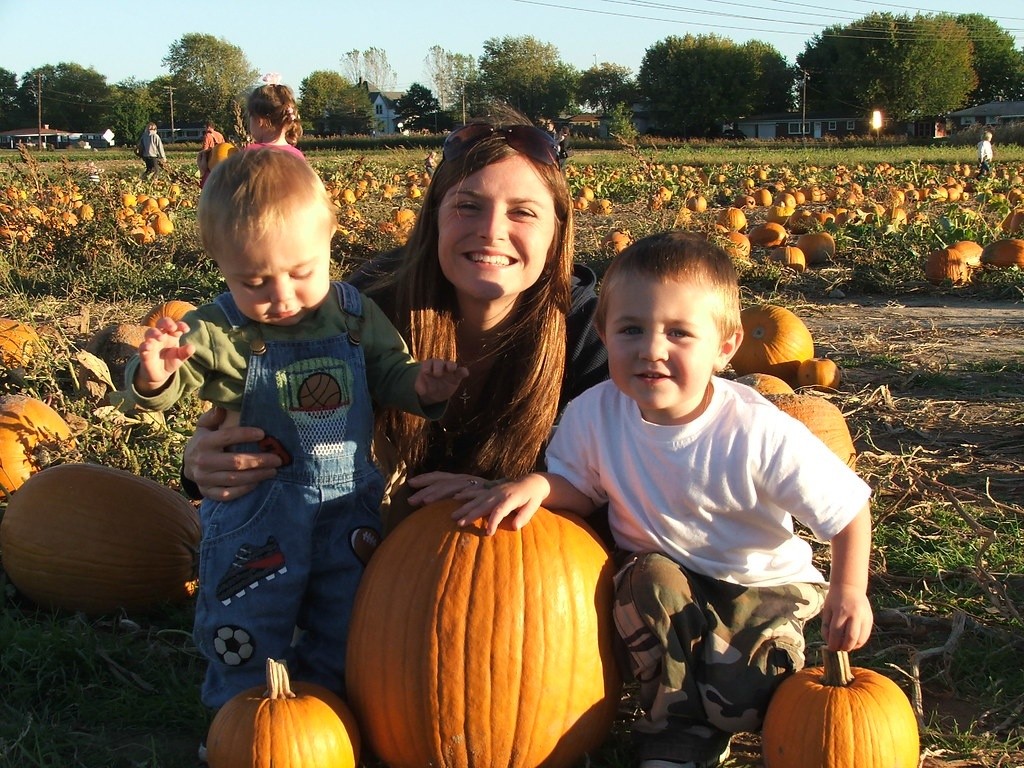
134 144 143 157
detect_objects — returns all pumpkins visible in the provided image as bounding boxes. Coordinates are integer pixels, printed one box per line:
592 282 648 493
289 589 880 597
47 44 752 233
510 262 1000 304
204 658 362 768
208 141 237 173
343 497 623 767
141 301 198 328
0 179 192 246
328 171 431 245
760 644 920 768
567 164 1024 288
721 304 856 469
0 394 80 506
0 317 42 371
0 463 204 620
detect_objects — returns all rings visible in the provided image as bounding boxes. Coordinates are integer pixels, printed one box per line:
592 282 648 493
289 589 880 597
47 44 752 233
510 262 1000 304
469 478 478 486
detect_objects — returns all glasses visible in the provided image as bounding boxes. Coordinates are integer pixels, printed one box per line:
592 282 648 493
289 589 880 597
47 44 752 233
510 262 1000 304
443 123 561 171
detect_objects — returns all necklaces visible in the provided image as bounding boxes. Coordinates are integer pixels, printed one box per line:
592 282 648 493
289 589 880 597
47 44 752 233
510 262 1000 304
458 364 494 399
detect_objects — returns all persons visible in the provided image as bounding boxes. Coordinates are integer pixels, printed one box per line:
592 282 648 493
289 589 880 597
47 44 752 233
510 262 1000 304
202 120 225 152
451 231 875 768
198 85 306 187
535 116 570 167
139 122 166 181
977 132 993 180
119 147 467 762
180 109 610 532
